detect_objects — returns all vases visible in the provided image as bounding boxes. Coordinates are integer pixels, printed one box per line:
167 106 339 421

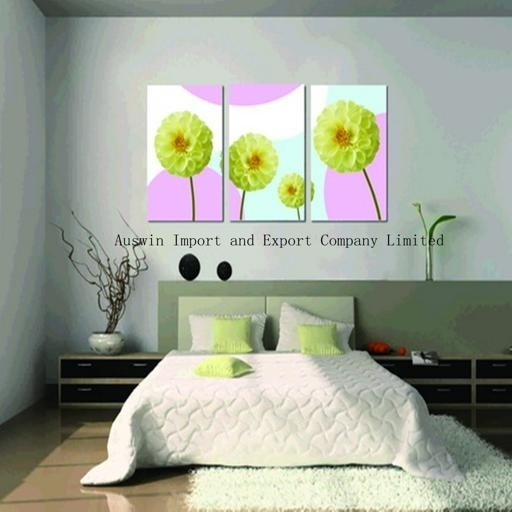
86 331 127 355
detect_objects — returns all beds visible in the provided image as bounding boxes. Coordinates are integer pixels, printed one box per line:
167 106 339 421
79 295 467 487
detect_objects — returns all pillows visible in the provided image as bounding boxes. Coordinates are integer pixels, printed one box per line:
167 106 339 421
188 302 355 378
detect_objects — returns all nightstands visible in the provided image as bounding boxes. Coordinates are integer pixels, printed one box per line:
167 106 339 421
369 354 512 435
58 353 164 445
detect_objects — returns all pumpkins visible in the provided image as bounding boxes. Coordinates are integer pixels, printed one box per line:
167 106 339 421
368 343 390 353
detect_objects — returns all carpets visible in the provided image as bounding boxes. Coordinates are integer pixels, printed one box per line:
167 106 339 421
186 415 512 512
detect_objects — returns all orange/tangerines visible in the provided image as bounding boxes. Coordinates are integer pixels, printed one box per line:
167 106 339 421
399 347 405 355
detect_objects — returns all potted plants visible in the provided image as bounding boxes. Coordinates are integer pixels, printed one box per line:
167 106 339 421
412 202 456 282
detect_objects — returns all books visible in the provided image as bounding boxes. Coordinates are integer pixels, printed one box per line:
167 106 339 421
411 349 440 367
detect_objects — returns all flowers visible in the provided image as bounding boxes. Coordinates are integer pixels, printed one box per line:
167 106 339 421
154 110 213 222
278 173 305 221
229 133 279 220
312 99 382 220
